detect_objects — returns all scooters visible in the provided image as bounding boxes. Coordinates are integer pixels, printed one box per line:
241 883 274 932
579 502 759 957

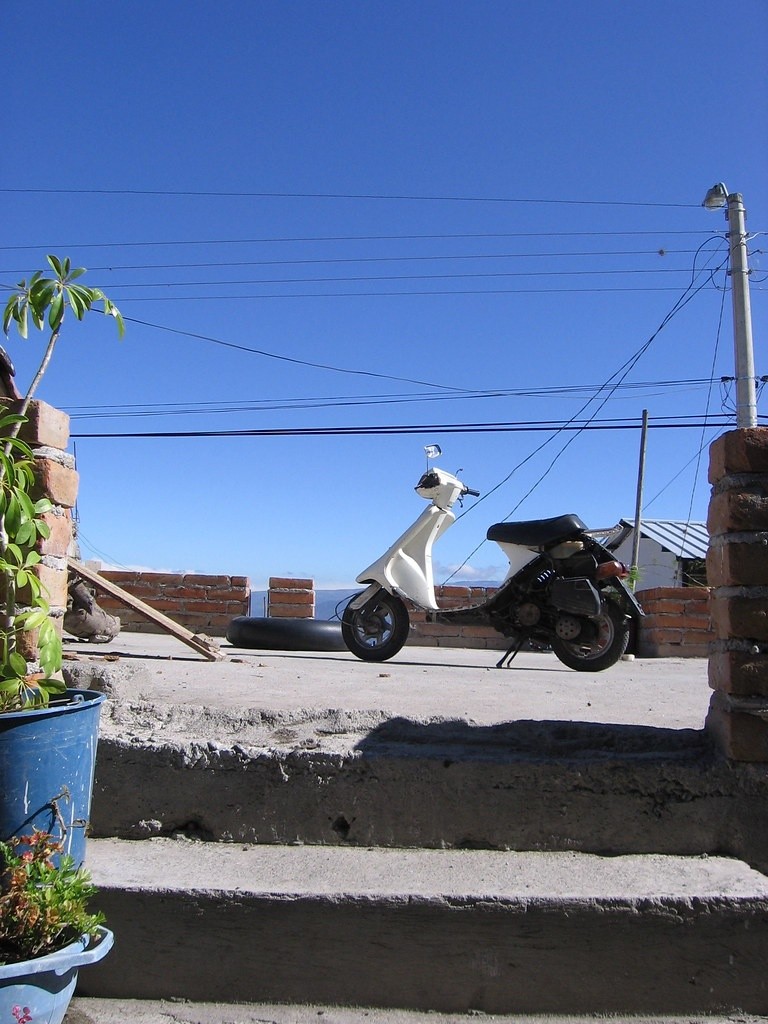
337 444 647 670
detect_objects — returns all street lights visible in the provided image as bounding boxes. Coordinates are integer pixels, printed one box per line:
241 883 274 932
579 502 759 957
702 184 759 431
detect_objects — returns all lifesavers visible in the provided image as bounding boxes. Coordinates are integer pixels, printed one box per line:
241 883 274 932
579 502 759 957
227 615 352 652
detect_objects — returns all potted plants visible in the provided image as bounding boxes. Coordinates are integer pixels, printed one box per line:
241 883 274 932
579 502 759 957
1 253 116 879
591 566 640 654
1 787 115 1024
